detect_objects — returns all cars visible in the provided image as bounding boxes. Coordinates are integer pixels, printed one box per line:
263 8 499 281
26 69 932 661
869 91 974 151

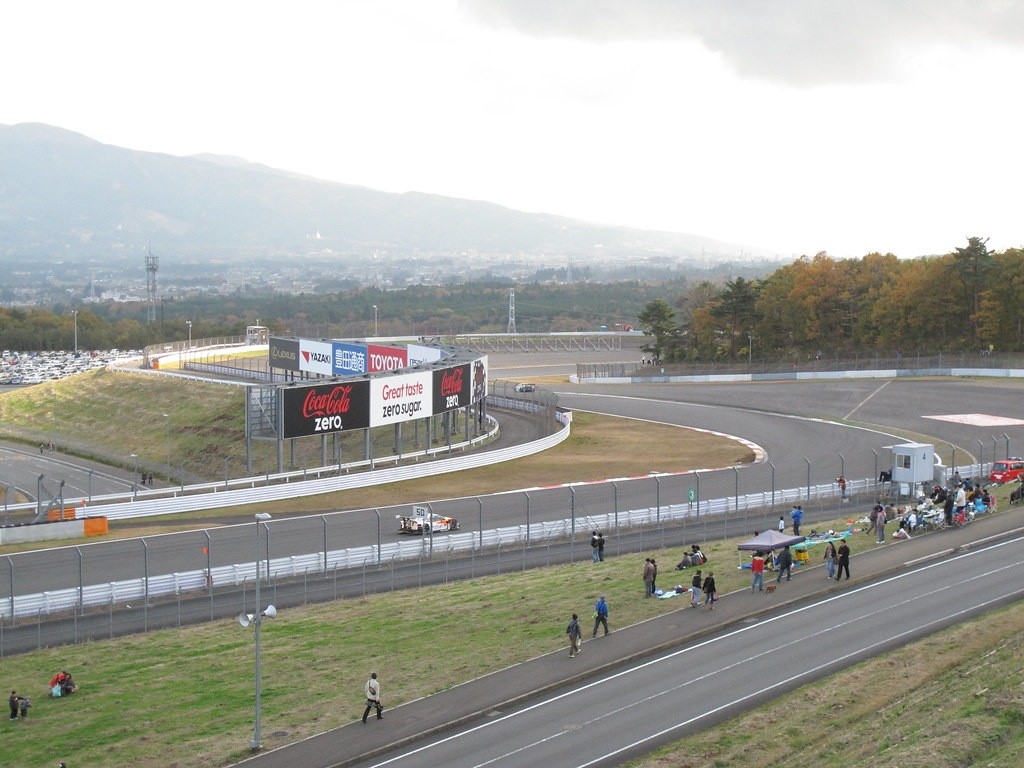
0 345 200 385
515 383 535 393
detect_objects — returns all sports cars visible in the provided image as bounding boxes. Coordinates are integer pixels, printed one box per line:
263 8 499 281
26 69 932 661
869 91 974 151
395 512 460 536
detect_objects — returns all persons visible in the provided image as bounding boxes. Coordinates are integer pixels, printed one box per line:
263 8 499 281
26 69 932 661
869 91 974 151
750 505 850 591
592 594 610 637
988 342 994 355
865 472 990 544
642 558 657 598
50 671 75 698
838 476 846 496
677 545 707 570
59 762 66 768
566 613 582 658
363 673 384 723
9 690 32 721
40 439 54 453
641 355 657 365
591 531 604 562
690 570 716 608
142 471 153 485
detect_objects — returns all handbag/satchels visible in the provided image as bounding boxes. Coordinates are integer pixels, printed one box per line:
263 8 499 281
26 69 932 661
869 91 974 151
369 681 376 695
52 685 61 697
691 589 694 600
592 611 598 618
713 592 719 601
578 639 581 646
704 587 708 593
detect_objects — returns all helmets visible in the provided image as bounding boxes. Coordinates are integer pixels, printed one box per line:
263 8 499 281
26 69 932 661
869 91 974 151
934 486 941 493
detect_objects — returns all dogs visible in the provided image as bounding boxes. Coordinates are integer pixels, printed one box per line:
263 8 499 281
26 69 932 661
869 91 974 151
765 585 776 594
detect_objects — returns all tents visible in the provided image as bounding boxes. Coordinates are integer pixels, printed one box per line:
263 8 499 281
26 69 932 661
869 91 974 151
738 529 806 570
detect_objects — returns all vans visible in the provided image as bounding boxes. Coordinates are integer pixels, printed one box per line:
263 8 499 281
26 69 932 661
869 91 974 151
989 456 1024 485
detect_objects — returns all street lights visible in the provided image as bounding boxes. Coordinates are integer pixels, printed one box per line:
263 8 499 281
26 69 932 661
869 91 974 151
238 513 278 752
186 320 192 351
373 304 378 336
74 310 78 352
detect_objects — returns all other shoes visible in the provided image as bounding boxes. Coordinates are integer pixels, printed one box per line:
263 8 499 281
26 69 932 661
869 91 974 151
835 577 839 580
576 649 580 653
828 575 835 579
569 654 574 658
845 575 850 580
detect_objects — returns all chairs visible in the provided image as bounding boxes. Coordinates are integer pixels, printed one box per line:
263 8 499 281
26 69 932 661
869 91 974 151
983 496 998 514
912 516 946 535
